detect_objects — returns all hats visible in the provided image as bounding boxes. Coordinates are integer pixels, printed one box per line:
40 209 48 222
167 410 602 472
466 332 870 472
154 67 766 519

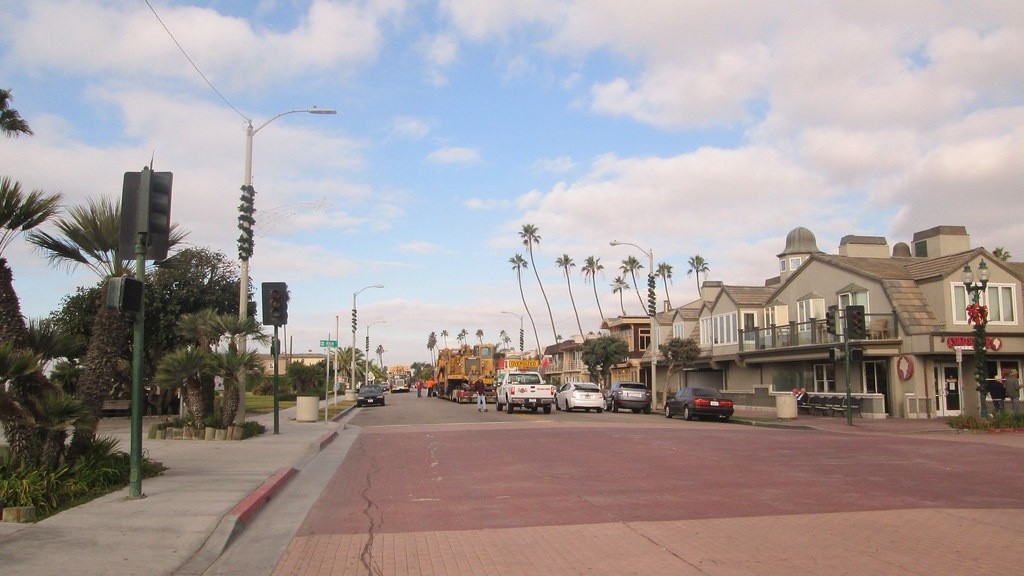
1010 369 1018 376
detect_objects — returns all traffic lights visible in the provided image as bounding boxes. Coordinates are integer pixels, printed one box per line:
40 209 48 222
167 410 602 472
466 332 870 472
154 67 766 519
262 282 287 325
829 348 837 361
825 305 840 334
851 350 863 361
845 306 867 340
118 275 146 314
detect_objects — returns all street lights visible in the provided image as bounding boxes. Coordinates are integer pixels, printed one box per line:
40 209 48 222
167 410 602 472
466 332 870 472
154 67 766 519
962 258 991 419
231 107 338 423
500 311 524 360
350 284 384 390
609 240 658 413
365 321 387 385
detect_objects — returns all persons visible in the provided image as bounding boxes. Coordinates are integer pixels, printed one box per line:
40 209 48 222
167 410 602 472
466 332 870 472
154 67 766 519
427 378 433 397
1005 369 1024 413
791 388 799 398
416 378 423 397
797 388 808 405
475 380 488 412
985 375 1006 418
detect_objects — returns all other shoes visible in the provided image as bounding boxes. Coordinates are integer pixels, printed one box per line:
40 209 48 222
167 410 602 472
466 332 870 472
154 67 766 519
484 409 488 412
989 414 994 418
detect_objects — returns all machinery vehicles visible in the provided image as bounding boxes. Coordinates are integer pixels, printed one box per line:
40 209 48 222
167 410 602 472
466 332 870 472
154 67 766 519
429 343 499 404
386 365 412 393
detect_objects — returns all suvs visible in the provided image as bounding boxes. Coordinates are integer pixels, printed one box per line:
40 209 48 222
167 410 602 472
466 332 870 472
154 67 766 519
603 381 652 415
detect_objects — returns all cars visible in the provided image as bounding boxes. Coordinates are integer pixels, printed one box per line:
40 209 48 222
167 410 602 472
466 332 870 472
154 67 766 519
664 387 735 423
554 382 604 413
354 383 386 408
377 382 389 392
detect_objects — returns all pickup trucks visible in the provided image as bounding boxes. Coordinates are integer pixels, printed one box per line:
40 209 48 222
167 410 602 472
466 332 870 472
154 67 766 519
496 371 557 414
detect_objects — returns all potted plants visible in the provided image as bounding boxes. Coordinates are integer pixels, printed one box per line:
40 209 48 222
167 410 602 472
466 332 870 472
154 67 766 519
295 389 321 422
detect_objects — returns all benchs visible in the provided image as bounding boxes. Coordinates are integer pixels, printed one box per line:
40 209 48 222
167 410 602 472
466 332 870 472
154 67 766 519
866 319 890 338
101 399 132 419
799 396 864 420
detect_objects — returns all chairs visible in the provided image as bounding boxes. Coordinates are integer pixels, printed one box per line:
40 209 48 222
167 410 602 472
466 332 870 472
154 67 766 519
510 376 516 382
